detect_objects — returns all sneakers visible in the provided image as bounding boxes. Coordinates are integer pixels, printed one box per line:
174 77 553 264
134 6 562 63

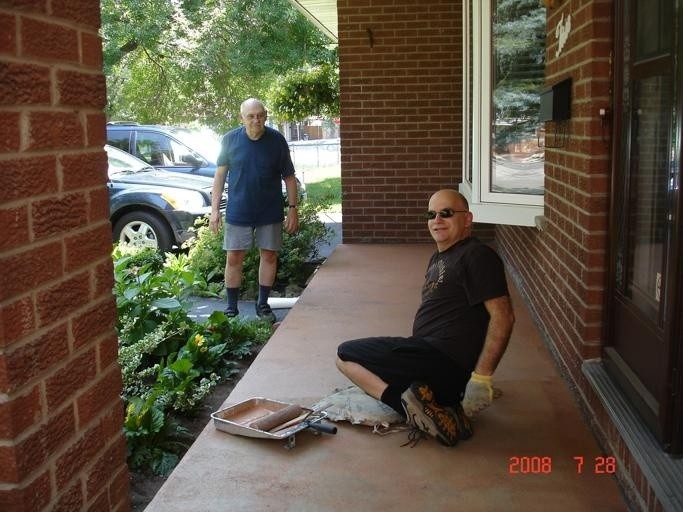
401 382 472 446
224 309 239 317
255 303 276 322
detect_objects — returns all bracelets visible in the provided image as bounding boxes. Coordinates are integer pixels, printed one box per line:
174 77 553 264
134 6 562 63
288 203 297 211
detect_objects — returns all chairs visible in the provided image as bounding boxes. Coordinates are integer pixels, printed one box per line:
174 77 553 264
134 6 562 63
120 138 150 164
150 142 174 166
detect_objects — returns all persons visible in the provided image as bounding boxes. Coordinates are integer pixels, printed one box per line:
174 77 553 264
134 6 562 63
207 96 297 325
333 188 516 447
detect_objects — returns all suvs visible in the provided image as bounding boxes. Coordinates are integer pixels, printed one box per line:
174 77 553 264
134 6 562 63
106 117 308 212
104 142 226 265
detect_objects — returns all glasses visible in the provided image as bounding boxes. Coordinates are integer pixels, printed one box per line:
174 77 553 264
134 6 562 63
424 209 466 219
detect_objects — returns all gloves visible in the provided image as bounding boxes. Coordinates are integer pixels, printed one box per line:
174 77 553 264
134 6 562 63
462 372 493 416
